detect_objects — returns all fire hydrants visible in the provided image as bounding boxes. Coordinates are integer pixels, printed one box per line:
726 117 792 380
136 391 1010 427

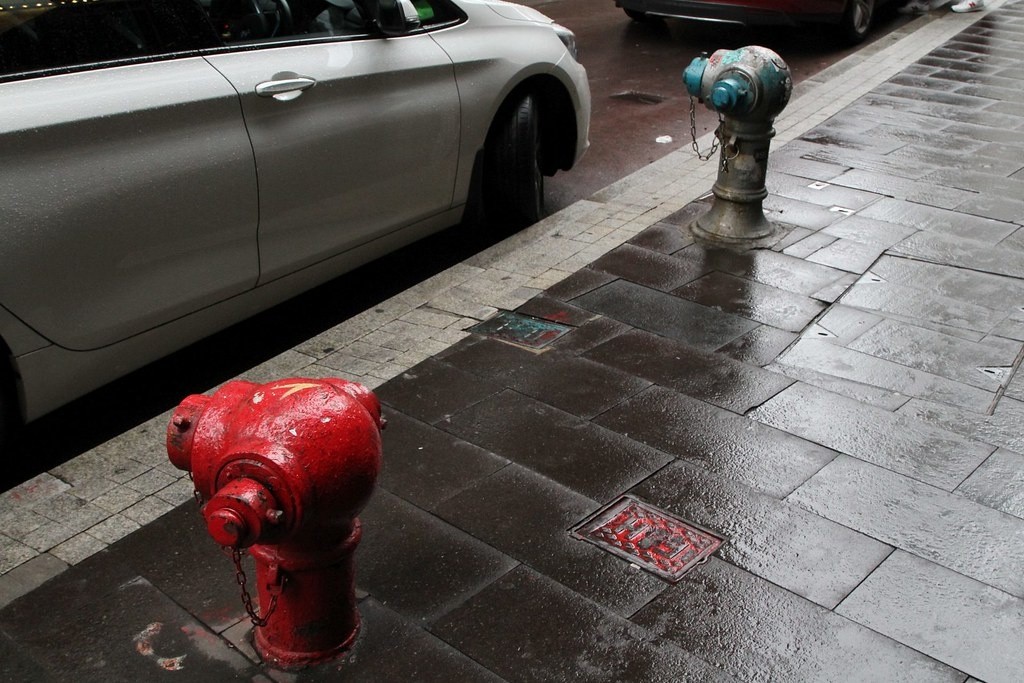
164 375 383 672
681 42 792 241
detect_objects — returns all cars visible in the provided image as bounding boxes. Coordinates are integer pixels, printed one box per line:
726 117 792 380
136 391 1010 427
621 1 927 45
0 2 595 430
